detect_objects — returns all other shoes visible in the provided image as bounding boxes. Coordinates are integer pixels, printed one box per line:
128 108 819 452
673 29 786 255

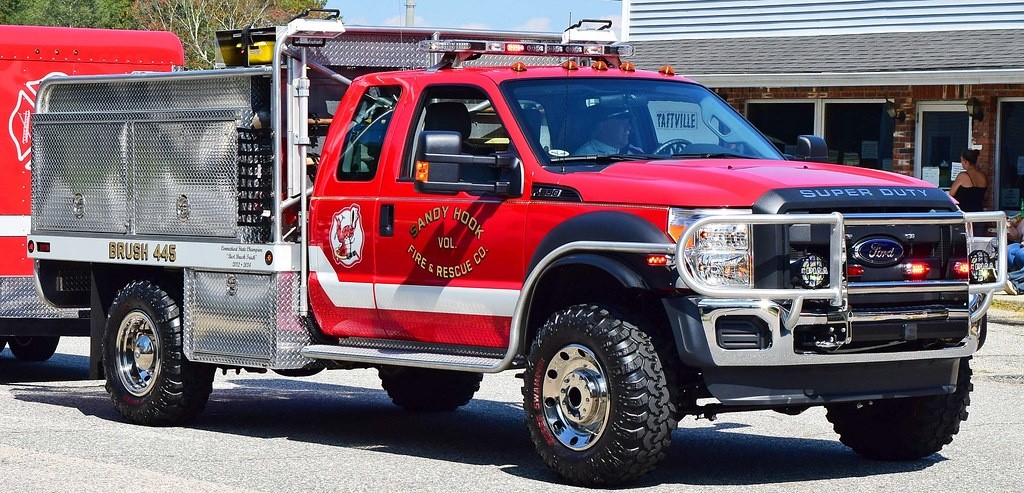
1003 280 1020 296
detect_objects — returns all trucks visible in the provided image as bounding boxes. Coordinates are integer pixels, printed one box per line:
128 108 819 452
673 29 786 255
27 6 1010 490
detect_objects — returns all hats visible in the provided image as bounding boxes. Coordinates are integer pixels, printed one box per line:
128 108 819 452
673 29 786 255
593 102 637 120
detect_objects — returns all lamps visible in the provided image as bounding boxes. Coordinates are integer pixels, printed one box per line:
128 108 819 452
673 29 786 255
883 96 906 123
964 97 983 123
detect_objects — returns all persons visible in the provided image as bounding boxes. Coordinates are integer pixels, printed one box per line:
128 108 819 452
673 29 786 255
570 100 643 156
949 150 988 236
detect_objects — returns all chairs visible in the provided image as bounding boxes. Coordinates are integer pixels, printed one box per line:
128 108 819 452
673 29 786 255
424 101 494 182
566 108 591 148
